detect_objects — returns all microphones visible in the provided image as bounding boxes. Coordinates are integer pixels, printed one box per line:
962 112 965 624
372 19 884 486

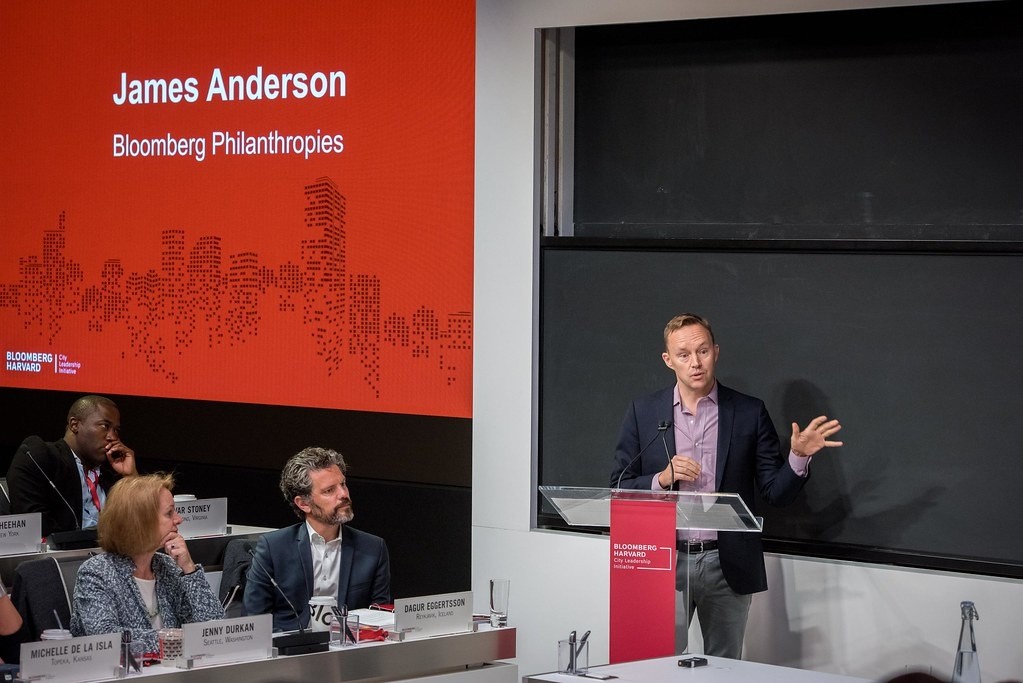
243 541 330 655
611 420 674 501
20 443 100 549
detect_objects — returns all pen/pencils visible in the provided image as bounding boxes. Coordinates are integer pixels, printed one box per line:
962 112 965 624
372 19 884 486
570 631 576 673
123 630 131 673
567 630 591 671
121 636 140 673
331 606 357 644
340 604 348 644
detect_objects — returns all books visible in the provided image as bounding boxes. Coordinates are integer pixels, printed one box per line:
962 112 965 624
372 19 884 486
333 607 395 631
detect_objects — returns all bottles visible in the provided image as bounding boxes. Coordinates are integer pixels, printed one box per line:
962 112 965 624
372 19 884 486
951 602 983 683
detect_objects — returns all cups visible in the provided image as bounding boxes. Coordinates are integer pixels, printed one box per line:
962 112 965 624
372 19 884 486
120 640 143 676
329 614 359 648
158 629 182 666
308 597 338 639
558 638 589 676
173 495 196 501
488 578 510 628
40 629 73 641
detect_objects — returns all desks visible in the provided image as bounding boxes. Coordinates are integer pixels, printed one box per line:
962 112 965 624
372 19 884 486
0 525 517 683
523 651 871 683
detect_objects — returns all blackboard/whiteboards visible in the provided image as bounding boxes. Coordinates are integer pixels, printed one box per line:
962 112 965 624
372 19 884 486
529 235 1023 580
561 1 1021 238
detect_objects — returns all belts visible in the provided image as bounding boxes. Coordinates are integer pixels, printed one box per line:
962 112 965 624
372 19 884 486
676 540 719 554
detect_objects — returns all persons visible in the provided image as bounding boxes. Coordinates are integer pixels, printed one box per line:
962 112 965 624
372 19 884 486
70 471 224 661
607 311 844 661
244 446 392 635
7 395 142 550
0 579 23 665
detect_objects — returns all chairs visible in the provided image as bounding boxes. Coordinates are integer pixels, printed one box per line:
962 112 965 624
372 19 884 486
0 477 257 641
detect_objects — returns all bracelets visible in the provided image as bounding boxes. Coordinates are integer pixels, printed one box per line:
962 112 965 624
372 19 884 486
178 564 200 577
